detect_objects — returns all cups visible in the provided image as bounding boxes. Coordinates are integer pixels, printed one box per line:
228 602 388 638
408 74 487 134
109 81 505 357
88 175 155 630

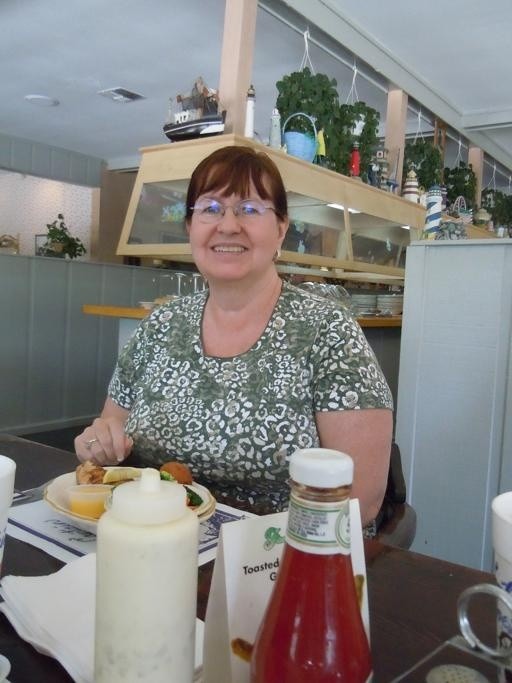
0 454 17 597
489 491 512 662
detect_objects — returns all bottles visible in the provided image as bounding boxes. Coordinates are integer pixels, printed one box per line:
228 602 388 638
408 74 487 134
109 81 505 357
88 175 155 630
138 270 210 310
92 465 199 681
247 446 375 681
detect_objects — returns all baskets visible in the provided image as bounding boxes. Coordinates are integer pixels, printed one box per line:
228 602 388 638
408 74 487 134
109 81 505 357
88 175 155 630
283 112 318 163
450 196 474 221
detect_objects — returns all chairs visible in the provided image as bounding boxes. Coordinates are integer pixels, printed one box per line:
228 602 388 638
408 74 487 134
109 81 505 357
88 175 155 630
376 443 418 550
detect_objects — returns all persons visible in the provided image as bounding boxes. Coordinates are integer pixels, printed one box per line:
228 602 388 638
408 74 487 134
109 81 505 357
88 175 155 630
74 147 394 539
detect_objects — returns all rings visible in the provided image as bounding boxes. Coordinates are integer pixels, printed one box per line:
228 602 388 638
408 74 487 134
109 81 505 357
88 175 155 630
84 438 98 450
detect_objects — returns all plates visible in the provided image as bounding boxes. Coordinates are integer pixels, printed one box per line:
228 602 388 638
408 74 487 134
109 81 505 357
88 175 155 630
349 289 404 319
41 463 217 529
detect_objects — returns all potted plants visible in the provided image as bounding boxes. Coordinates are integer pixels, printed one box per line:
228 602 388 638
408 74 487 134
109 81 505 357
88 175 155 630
46 213 87 260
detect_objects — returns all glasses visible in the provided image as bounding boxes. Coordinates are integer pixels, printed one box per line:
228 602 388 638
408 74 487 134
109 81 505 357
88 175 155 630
190 199 284 224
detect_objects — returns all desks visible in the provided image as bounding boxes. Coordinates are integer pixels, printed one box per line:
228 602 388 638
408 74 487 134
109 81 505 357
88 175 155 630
82 304 402 439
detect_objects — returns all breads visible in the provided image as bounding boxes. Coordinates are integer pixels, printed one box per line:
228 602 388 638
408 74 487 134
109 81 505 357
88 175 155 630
160 462 192 484
